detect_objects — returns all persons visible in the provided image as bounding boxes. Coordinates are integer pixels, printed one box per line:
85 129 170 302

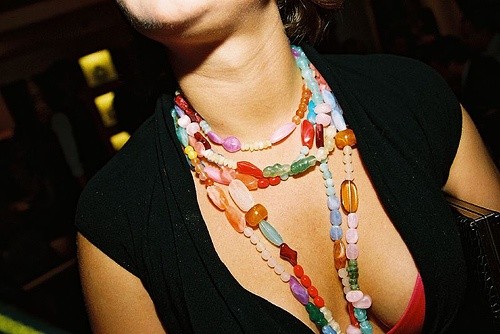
72 0 500 334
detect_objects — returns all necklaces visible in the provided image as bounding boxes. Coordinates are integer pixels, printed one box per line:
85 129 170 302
173 45 372 334
